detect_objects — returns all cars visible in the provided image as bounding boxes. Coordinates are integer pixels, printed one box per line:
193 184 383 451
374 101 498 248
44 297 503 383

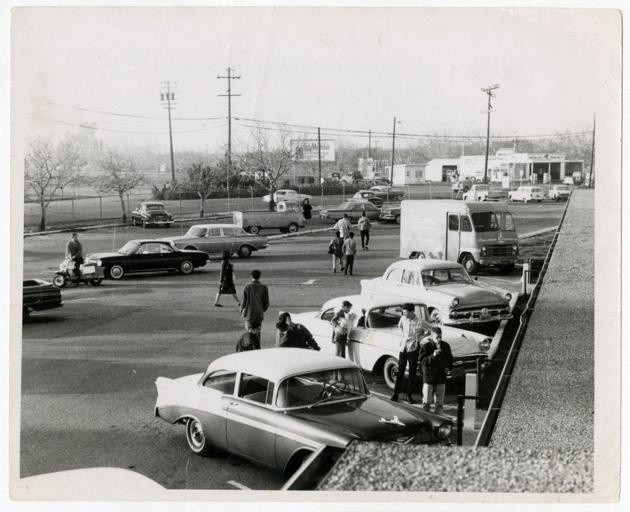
154 347 464 478
360 258 513 326
156 223 268 258
319 185 401 225
289 294 493 390
262 190 313 206
85 238 210 280
132 201 175 229
452 176 572 203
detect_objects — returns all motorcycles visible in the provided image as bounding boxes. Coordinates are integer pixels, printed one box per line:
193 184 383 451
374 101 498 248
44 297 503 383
53 257 106 287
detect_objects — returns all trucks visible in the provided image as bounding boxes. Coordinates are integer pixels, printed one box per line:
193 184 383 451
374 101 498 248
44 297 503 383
399 199 520 276
232 201 307 233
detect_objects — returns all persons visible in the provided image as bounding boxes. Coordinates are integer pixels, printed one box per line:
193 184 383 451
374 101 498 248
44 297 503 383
215 250 241 306
234 312 321 351
418 327 454 416
330 300 352 358
65 233 83 284
333 312 356 343
392 303 432 405
301 197 313 230
270 194 276 213
239 269 269 341
328 210 371 275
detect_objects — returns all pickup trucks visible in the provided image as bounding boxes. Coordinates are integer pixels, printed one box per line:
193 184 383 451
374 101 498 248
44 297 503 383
23 279 63 322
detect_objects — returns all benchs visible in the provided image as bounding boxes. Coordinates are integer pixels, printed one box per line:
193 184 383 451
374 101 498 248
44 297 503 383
206 376 305 398
243 384 325 407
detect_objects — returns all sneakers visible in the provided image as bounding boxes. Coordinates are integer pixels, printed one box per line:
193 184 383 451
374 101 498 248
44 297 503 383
391 395 398 401
406 396 413 404
